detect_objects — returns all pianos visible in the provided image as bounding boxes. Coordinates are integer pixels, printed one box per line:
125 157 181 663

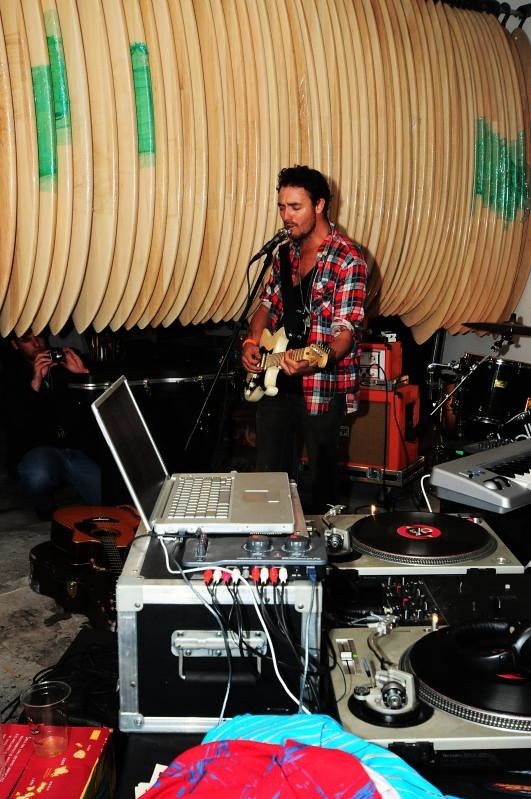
430 438 530 514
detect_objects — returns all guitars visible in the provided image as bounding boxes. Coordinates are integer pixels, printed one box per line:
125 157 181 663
244 326 332 403
51 506 141 583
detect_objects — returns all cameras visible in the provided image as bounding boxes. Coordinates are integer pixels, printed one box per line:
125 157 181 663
46 348 69 367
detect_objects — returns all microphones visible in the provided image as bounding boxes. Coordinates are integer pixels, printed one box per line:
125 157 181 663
253 226 291 262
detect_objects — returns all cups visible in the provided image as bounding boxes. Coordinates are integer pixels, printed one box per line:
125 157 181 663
20 681 74 759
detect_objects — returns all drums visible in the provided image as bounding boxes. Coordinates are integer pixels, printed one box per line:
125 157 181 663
431 373 460 433
147 373 223 411
454 351 531 425
69 374 149 414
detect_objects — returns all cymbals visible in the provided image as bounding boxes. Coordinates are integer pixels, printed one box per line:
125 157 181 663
462 321 531 335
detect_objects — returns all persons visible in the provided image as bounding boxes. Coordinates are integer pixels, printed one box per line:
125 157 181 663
242 165 370 514
6 328 121 525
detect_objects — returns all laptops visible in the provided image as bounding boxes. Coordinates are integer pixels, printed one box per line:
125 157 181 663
93 374 297 536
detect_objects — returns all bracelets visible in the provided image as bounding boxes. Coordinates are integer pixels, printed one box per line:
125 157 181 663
242 338 256 348
